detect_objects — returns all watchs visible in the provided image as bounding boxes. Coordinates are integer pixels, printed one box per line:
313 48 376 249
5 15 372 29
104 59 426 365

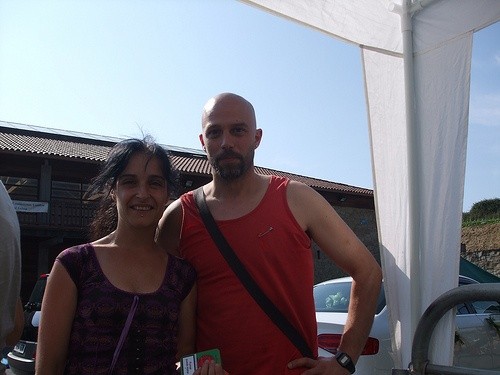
334 352 355 374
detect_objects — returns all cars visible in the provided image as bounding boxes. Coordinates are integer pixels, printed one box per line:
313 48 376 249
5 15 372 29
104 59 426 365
313 271 500 374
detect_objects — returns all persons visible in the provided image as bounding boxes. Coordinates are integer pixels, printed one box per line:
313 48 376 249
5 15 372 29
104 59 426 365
35 135 230 375
154 92 382 375
0 180 26 375
